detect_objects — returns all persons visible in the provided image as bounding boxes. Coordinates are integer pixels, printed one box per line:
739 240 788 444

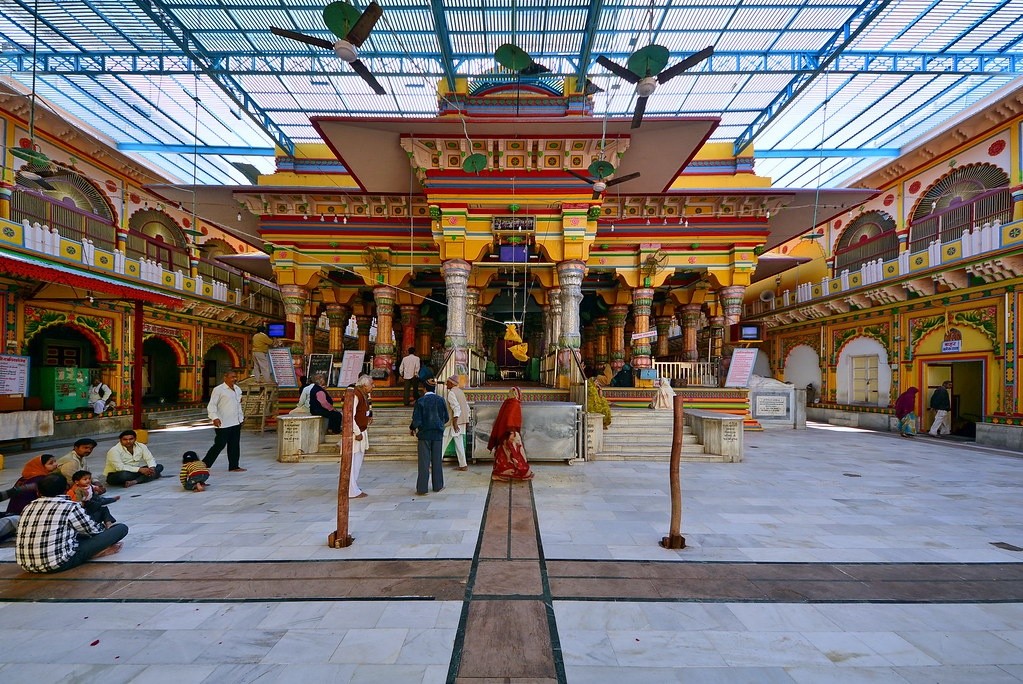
88 377 112 417
0 438 128 574
252 325 277 383
399 347 420 408
180 451 210 492
927 380 952 438
419 360 434 383
289 372 368 435
488 386 535 482
429 375 470 472
103 430 164 488
339 374 374 499
201 367 248 472
409 378 450 496
894 387 919 438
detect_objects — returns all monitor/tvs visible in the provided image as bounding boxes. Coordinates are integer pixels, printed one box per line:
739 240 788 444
267 322 286 338
739 323 760 340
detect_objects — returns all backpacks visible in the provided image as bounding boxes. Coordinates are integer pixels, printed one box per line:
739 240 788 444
930 386 941 408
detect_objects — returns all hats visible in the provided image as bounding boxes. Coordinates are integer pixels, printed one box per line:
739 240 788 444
448 375 459 385
424 379 437 391
182 451 199 464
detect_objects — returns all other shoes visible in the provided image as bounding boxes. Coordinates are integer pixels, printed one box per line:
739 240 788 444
900 433 914 438
228 467 247 472
91 542 123 558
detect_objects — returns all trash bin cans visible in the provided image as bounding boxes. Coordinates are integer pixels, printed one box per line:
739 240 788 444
528 357 540 381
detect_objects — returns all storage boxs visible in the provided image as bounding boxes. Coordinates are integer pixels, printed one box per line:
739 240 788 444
632 367 671 388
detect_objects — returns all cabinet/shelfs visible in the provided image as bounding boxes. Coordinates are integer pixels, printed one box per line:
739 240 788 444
240 383 279 438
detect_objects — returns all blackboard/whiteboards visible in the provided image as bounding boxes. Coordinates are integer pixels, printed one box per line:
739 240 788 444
307 353 333 386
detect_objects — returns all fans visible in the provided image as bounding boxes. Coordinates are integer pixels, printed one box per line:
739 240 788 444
269 0 387 95
0 0 56 190
362 246 390 284
634 246 669 288
596 0 713 129
567 116 640 200
